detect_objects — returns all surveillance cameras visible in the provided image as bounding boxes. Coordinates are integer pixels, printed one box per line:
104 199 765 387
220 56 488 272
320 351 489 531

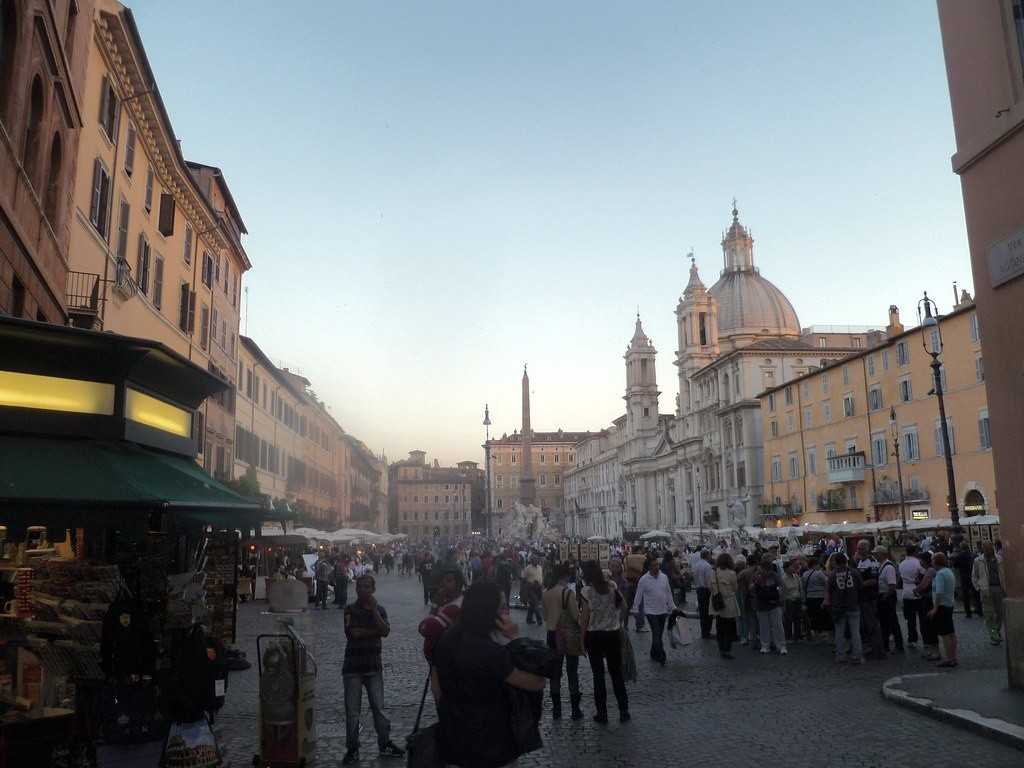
927 389 935 395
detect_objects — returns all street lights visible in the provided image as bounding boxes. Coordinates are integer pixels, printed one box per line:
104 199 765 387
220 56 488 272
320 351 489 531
695 466 703 543
889 405 908 539
917 290 964 601
483 404 492 542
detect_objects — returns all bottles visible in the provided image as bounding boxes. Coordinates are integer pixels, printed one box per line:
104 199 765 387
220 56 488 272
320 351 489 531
26 526 47 550
0 675 12 694
16 543 26 568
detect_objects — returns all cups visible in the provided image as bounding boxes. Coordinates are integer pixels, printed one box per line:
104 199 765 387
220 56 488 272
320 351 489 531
4 600 17 614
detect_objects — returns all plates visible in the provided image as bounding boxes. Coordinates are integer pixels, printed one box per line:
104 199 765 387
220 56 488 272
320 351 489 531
25 549 55 555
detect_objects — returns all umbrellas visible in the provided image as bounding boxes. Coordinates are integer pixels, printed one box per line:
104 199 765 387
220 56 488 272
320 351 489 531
714 515 999 550
220 528 382 543
639 530 671 541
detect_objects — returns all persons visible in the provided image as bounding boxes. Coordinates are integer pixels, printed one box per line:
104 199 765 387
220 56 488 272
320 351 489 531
563 536 579 544
418 569 464 709
430 579 545 768
373 536 559 625
687 535 1003 667
342 575 406 764
240 564 256 579
539 544 681 724
613 537 625 547
273 563 298 579
310 550 348 610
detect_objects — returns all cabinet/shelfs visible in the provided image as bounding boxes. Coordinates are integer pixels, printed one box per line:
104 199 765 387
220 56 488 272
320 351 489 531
9 528 245 717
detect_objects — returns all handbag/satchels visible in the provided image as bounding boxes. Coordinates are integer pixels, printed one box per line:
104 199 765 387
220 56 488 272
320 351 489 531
102 671 171 744
555 587 583 657
828 607 846 622
609 580 623 609
667 616 694 649
711 592 726 612
406 721 447 768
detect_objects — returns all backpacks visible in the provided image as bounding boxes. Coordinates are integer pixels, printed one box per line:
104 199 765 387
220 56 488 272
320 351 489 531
171 622 229 724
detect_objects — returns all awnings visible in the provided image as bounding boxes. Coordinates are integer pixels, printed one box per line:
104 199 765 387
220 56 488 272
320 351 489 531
0 435 268 531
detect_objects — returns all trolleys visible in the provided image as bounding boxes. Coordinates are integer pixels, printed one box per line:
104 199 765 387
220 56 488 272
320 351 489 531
251 634 320 768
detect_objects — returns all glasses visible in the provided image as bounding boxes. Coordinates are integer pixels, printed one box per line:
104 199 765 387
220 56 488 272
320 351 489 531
790 559 797 562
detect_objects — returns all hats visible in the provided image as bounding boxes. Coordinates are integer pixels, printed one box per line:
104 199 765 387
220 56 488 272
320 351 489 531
871 545 887 554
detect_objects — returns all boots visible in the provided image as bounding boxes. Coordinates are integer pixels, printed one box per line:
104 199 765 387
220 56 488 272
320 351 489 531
549 691 562 719
570 689 584 719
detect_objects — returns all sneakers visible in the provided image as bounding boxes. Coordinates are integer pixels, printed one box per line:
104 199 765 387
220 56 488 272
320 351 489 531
343 747 361 765
380 740 405 757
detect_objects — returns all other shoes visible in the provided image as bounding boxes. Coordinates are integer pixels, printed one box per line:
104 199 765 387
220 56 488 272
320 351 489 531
650 653 666 665
701 628 958 667
526 620 536 624
313 594 347 610
965 606 984 617
538 618 543 625
990 632 1003 645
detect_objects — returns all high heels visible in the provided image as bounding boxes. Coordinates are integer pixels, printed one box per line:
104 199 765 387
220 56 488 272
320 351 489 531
593 712 609 723
619 709 631 722
